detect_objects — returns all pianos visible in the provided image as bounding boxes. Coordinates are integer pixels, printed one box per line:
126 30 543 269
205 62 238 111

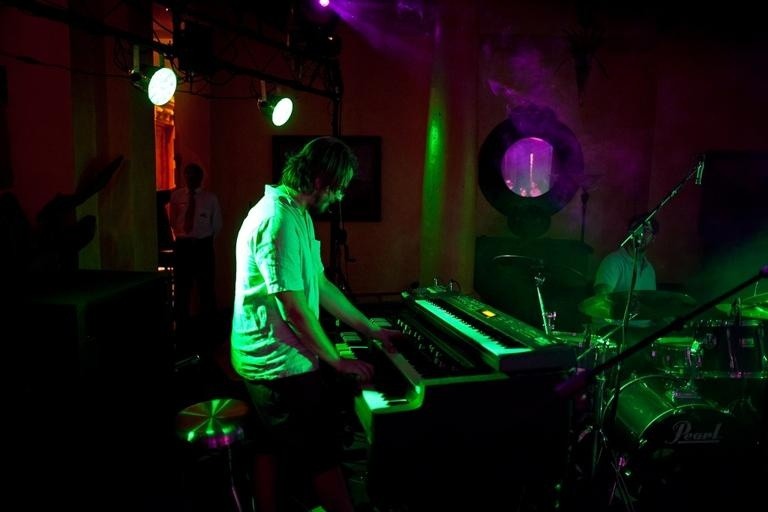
336 284 576 418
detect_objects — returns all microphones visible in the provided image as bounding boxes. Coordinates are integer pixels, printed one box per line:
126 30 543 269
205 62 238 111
695 151 706 185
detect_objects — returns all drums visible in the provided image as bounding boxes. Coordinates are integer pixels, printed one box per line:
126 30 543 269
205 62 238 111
705 317 768 380
600 374 739 483
554 332 617 376
646 332 706 373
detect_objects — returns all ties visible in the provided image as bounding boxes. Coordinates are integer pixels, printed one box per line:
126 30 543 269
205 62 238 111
182 191 197 234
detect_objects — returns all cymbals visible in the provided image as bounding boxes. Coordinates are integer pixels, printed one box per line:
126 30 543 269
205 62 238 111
493 253 585 283
715 303 768 319
579 291 698 320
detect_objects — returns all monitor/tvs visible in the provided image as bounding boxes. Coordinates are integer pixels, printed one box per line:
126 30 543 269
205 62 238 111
271 134 382 222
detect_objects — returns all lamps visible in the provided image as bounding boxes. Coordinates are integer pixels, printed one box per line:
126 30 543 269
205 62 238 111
254 80 295 128
127 39 177 108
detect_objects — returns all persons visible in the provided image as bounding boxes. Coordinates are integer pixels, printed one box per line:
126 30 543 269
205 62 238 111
170 163 223 333
228 135 401 512
594 213 659 295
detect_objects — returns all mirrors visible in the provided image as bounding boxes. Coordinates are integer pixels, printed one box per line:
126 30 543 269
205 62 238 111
498 136 558 199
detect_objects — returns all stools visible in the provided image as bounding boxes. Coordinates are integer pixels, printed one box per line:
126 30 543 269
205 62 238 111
172 397 259 511
